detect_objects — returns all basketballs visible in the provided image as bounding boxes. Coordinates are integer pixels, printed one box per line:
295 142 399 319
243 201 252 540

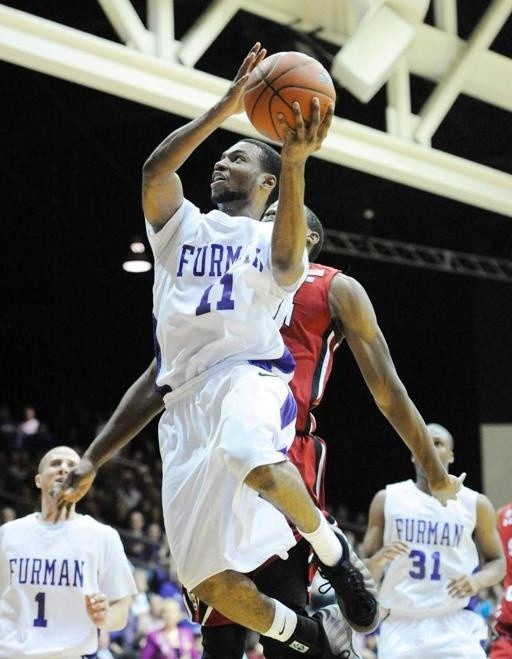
243 52 336 144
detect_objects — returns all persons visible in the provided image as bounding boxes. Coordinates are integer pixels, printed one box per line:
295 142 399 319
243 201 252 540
310 426 512 659
1 409 202 659
44 197 469 657
140 43 382 655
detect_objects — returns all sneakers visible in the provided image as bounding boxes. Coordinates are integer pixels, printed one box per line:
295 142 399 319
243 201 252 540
311 524 381 659
